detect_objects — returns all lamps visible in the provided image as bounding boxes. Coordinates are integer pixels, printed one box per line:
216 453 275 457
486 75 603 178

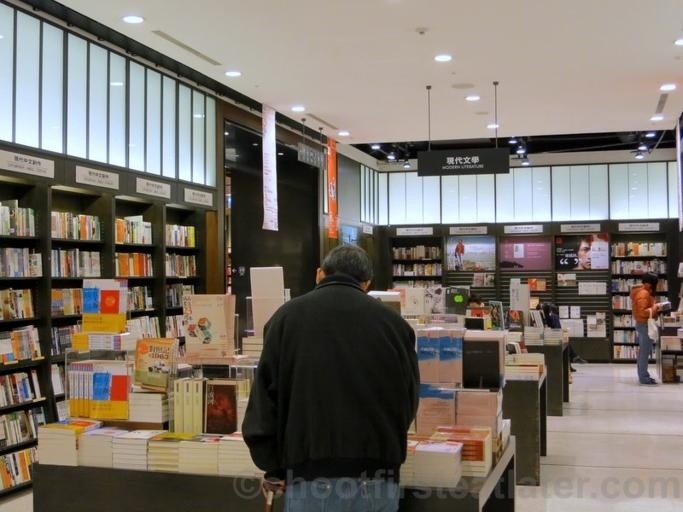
387 146 411 168
508 137 529 166
634 132 656 159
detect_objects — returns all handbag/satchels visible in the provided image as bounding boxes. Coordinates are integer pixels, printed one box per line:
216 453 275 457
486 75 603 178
648 319 658 341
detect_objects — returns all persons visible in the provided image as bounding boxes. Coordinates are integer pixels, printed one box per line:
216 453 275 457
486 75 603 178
242 244 420 511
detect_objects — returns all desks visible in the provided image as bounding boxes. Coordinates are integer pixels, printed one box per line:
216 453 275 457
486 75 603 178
28 330 570 512
656 327 683 383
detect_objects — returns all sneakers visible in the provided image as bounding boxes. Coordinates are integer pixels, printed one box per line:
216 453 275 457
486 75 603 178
576 357 587 363
571 368 576 372
642 377 658 384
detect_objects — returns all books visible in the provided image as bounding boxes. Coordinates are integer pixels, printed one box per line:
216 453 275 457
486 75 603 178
0 199 683 491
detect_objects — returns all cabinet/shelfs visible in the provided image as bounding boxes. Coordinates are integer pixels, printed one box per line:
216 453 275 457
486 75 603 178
378 218 683 363
0 140 220 495
